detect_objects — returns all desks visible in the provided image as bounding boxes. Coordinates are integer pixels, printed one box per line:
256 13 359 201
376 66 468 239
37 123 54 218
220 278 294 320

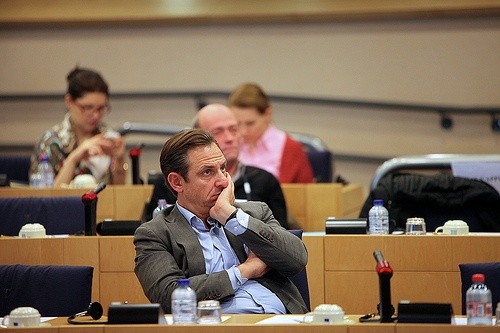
0 184 500 332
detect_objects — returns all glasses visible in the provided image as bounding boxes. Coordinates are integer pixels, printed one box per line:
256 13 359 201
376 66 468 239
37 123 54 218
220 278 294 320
73 97 112 118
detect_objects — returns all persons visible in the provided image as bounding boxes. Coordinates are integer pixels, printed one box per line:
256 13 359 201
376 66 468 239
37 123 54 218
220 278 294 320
134 128 308 314
226 82 311 184
29 66 128 187
141 103 288 229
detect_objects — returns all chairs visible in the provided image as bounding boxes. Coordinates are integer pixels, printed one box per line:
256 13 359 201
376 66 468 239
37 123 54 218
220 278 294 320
0 154 32 186
291 134 334 182
0 196 88 235
288 230 312 313
0 264 93 317
458 262 500 315
358 173 500 231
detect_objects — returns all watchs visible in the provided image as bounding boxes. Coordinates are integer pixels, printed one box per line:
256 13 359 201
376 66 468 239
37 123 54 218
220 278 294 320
112 163 128 175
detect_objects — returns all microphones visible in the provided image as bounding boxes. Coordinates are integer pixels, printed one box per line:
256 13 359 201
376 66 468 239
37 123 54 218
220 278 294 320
374 250 393 277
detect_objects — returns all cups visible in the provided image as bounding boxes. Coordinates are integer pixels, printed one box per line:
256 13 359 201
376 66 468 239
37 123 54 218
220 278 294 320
303 303 344 326
197 300 222 325
2 307 41 327
496 303 500 325
69 174 94 189
19 223 46 239
435 220 468 235
406 218 426 235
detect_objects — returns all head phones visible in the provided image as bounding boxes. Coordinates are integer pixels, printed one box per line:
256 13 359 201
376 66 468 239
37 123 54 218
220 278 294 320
67 302 108 325
358 301 398 321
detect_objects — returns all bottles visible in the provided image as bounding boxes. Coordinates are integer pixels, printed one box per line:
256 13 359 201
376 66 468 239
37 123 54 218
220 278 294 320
466 274 492 325
368 200 389 234
171 279 197 324
153 199 169 218
38 156 53 187
